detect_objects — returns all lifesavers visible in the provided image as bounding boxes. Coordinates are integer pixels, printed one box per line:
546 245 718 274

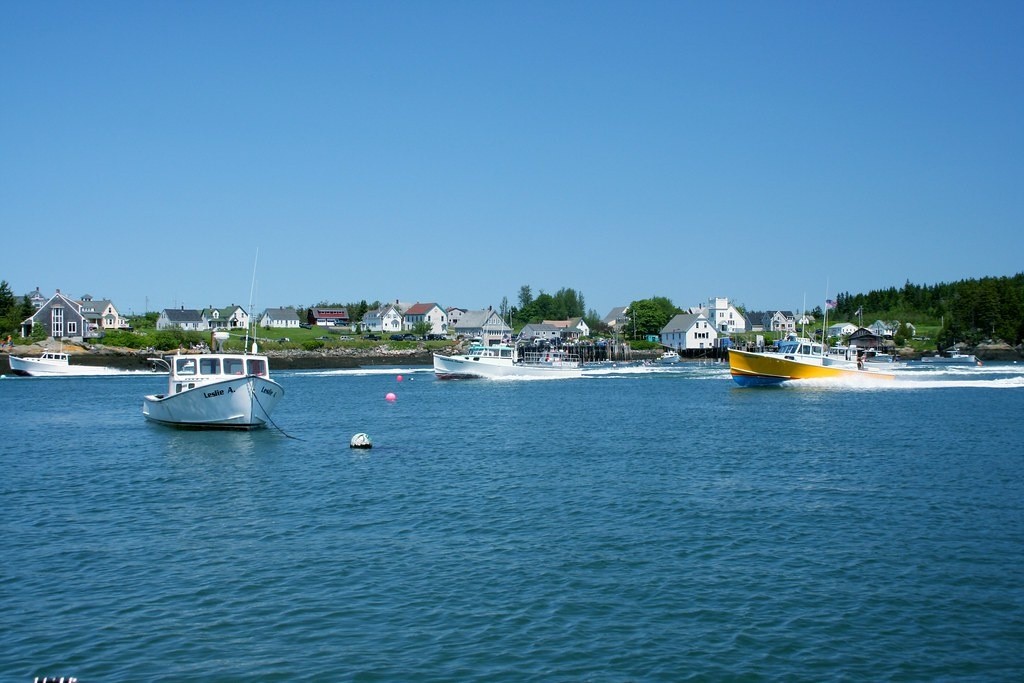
469 349 474 355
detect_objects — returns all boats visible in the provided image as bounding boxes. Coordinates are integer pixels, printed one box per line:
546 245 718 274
540 349 579 369
8 350 111 378
431 341 579 379
659 351 680 364
921 349 977 363
726 307 895 387
142 351 284 430
773 338 902 366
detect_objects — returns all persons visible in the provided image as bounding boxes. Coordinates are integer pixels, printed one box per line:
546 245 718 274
1 335 13 348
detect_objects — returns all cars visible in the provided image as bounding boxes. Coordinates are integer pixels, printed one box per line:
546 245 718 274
388 331 446 341
239 336 254 342
361 334 383 341
315 336 332 342
276 337 291 345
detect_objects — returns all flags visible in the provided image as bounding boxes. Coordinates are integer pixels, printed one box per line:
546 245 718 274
825 299 837 309
855 308 862 317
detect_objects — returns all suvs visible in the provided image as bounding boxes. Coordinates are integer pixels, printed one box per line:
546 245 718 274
339 336 356 341
